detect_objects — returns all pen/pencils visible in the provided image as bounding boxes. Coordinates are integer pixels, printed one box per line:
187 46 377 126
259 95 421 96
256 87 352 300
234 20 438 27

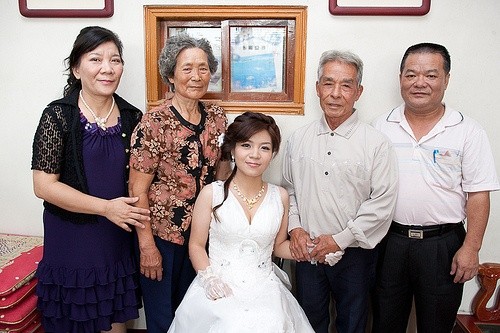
433 149 440 165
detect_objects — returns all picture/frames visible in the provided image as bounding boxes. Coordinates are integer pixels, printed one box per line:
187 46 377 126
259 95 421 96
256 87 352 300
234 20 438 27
19 0 114 18
329 0 432 16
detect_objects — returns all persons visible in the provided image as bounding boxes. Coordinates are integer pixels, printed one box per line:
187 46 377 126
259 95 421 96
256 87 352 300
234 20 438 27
377 44 497 333
164 110 345 333
32 25 153 333
129 35 233 333
280 52 400 333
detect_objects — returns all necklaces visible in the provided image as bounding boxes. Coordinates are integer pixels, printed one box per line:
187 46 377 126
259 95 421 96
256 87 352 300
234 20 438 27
234 176 265 209
79 89 115 131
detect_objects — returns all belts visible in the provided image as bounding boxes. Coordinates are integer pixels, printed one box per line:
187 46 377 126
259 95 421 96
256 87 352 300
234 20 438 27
391 220 463 240
